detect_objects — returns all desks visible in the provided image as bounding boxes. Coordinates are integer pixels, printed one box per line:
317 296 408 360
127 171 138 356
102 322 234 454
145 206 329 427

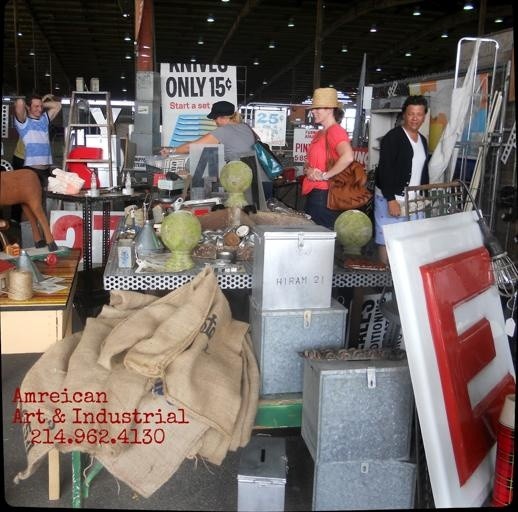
101 214 393 350
1 248 82 355
45 187 146 325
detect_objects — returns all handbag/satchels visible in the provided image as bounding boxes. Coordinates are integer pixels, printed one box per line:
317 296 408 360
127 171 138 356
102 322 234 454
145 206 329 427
243 122 284 179
325 127 373 211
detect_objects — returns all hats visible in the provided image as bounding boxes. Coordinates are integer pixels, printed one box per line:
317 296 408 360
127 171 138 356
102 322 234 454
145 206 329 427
207 100 234 120
304 87 343 109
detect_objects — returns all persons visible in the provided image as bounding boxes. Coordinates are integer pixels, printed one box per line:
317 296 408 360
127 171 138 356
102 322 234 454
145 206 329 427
157 101 269 212
13 94 63 216
10 92 55 224
299 87 355 230
373 94 431 265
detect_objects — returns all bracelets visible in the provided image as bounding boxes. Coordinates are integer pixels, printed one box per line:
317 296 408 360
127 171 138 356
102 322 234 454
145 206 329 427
313 167 328 181
169 147 176 155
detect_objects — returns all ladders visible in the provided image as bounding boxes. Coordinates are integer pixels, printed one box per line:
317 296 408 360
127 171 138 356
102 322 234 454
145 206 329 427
62 91 112 190
